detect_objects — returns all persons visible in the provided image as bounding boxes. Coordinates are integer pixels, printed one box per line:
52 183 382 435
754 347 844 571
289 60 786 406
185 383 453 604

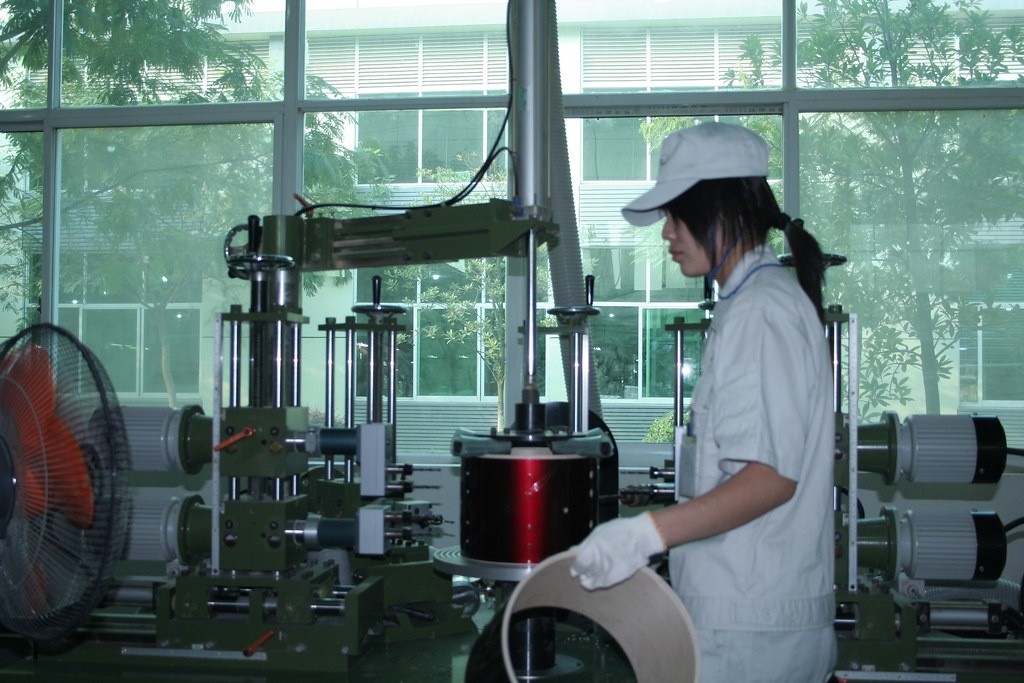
568 122 838 683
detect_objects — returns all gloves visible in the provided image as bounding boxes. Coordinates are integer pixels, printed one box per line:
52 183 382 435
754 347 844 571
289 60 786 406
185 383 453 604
569 509 668 592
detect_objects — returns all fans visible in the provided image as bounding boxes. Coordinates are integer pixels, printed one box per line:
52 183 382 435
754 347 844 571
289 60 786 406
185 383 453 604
0 324 134 641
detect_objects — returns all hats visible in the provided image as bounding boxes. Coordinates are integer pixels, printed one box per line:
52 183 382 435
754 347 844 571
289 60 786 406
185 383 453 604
622 122 767 226
